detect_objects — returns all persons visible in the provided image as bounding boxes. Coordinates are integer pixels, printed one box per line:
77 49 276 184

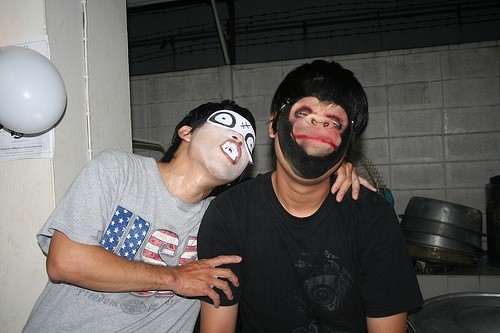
21 100 256 333
196 59 424 333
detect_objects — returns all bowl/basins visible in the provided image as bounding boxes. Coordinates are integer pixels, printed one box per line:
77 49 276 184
396 196 488 274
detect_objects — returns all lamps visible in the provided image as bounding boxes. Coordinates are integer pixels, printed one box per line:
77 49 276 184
0 46 68 139
